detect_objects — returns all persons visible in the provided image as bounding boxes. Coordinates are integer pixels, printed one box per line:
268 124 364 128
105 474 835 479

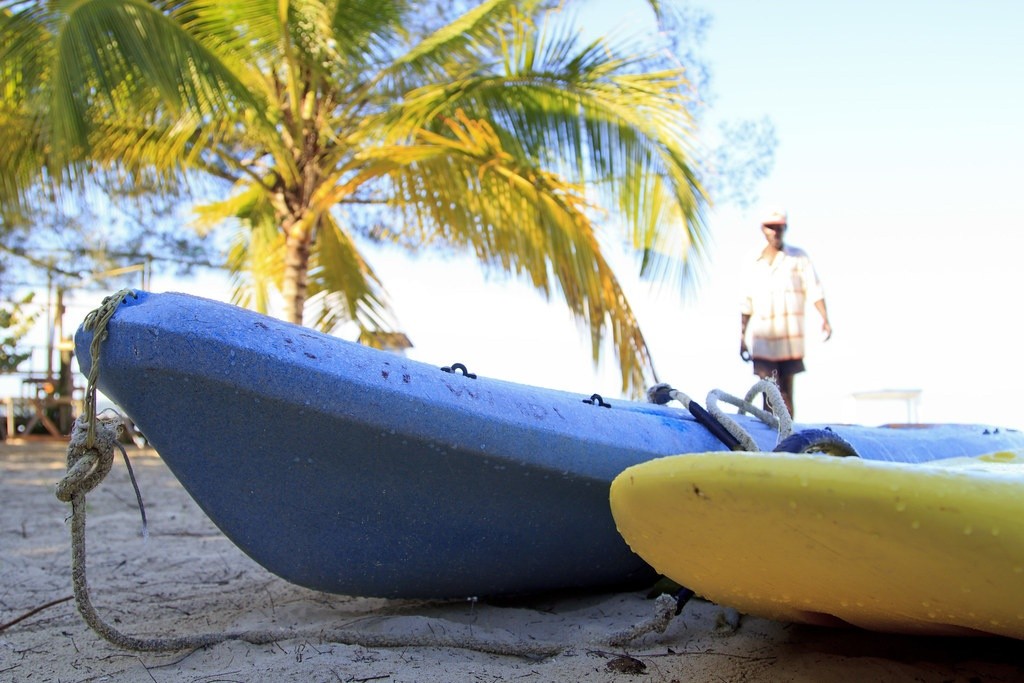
734 205 832 427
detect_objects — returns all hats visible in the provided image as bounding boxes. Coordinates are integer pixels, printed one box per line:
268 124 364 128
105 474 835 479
762 212 787 226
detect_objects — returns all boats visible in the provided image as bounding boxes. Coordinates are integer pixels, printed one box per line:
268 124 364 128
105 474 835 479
607 453 1023 640
76 288 1022 599
